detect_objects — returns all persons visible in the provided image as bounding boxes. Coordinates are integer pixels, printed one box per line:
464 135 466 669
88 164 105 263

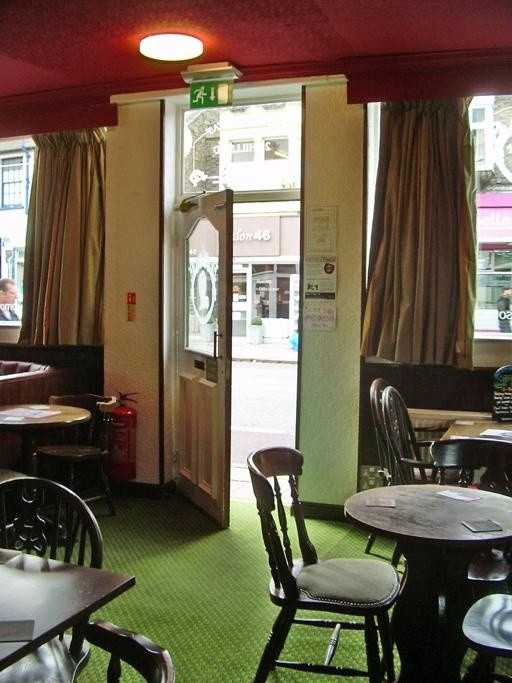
0 277 20 321
496 287 512 333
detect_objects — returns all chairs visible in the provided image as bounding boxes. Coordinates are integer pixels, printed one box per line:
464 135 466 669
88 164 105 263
248 447 400 682
37 394 120 515
461 592 510 682
364 376 511 658
0 476 175 683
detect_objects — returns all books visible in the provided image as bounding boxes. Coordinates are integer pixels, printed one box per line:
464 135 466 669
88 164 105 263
0 620 35 642
437 489 481 502
460 518 503 533
450 417 512 444
0 402 62 422
365 498 396 507
0 547 22 565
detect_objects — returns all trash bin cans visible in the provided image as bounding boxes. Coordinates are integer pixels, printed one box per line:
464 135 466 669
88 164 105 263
231 301 247 337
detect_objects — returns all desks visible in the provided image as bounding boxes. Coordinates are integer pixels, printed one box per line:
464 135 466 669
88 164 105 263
342 484 512 683
1 401 93 506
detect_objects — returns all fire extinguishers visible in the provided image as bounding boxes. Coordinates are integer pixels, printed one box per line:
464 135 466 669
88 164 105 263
111 391 138 479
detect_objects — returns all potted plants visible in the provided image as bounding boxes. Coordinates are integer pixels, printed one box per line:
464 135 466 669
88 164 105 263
247 316 264 343
